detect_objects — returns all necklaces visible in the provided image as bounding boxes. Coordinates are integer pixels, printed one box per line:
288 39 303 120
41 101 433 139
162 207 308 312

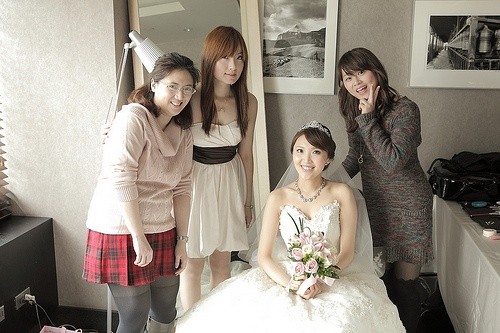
358 135 366 163
214 91 230 112
295 177 326 202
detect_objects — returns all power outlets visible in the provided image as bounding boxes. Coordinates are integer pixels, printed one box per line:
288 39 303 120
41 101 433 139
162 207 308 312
15 287 31 310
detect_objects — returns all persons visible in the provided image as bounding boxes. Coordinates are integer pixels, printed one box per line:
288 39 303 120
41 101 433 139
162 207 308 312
102 26 258 309
82 52 200 333
326 47 434 333
175 120 406 333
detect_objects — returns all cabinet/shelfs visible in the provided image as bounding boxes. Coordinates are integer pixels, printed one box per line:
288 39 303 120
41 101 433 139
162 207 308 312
0 215 59 333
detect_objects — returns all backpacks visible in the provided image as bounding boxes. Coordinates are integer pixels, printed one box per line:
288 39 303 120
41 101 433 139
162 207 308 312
426 151 500 176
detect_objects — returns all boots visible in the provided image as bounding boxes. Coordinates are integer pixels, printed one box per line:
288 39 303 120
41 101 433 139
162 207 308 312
147 314 177 333
381 269 421 333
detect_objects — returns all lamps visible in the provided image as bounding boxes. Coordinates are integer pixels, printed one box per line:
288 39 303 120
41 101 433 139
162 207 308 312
102 30 165 333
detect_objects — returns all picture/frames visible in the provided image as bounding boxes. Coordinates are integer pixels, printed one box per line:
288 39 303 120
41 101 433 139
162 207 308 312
257 0 339 95
408 0 500 90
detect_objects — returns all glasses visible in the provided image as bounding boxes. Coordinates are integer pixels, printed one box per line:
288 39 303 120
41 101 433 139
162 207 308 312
159 80 197 96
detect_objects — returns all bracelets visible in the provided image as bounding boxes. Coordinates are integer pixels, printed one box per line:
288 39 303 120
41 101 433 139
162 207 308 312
177 236 189 242
244 205 254 208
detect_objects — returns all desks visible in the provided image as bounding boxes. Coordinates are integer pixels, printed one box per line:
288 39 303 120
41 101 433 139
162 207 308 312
433 195 500 333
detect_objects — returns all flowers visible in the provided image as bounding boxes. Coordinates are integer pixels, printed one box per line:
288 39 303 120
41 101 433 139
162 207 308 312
287 211 342 296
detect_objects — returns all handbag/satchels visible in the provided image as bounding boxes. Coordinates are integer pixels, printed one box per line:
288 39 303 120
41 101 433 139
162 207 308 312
39 325 82 333
429 176 500 202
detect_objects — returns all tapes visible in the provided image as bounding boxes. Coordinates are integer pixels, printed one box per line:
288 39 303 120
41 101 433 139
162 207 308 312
483 229 496 236
